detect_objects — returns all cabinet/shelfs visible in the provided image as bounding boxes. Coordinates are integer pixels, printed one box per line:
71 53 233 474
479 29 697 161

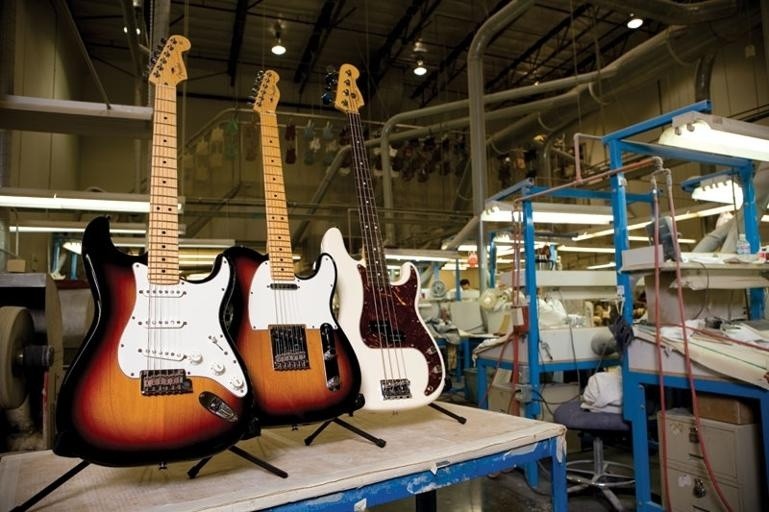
657 404 761 512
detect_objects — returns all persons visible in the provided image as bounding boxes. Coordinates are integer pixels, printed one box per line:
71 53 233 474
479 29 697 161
593 300 612 327
461 278 471 290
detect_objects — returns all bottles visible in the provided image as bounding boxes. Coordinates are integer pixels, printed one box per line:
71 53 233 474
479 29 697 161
535 255 550 270
736 234 751 258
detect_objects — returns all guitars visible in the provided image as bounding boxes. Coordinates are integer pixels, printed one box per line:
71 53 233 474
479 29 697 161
320 61 447 413
222 66 361 426
52 30 255 466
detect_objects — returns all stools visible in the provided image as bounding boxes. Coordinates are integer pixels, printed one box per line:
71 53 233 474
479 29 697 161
555 397 635 512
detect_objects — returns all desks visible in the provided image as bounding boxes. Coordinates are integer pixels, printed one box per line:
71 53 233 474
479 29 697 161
2 401 569 512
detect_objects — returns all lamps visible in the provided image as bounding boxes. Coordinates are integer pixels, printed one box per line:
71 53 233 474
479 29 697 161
269 0 287 57
1 186 185 217
478 111 769 223
624 1 644 30
410 21 430 77
7 216 187 237
66 237 234 251
